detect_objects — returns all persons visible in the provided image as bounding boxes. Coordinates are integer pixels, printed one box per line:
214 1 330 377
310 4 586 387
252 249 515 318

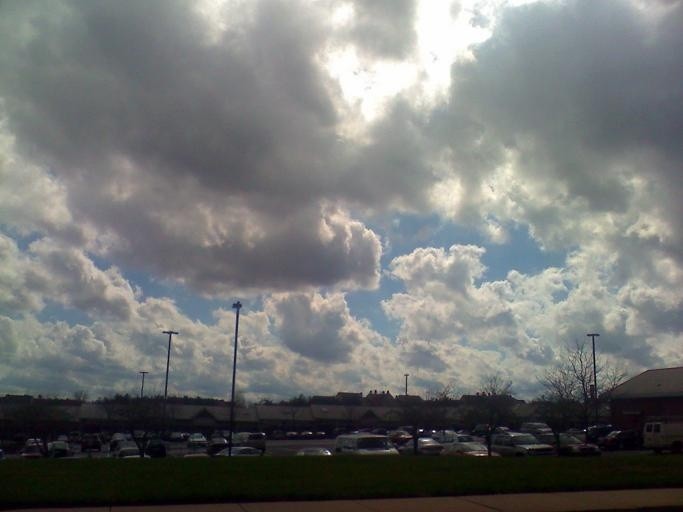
104 439 110 456
258 436 268 457
100 440 105 455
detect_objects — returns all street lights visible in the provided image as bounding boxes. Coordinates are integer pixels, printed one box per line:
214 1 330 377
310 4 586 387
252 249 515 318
162 331 178 418
403 373 409 395
139 372 148 400
586 333 599 431
228 301 241 456
